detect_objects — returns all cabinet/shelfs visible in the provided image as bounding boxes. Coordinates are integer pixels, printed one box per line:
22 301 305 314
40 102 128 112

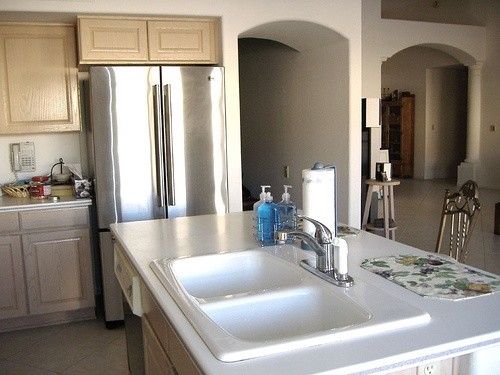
19 200 96 329
111 226 144 375
0 207 20 332
382 93 414 179
77 16 220 65
139 276 203 375
1 23 84 135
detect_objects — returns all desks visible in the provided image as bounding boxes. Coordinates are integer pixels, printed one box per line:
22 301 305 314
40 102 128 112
361 180 400 241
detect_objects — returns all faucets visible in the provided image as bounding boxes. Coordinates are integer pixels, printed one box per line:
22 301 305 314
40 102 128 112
287 214 333 273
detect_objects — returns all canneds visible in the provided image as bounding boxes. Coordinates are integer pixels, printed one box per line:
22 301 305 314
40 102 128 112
31 176 52 200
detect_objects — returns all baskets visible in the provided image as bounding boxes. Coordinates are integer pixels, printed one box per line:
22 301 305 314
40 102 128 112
1 180 45 198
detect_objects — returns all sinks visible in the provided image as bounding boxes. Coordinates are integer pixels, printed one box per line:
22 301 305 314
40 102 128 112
196 276 431 363
149 243 357 306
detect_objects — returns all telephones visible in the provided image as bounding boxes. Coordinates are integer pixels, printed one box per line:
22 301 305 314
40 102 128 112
10 142 36 186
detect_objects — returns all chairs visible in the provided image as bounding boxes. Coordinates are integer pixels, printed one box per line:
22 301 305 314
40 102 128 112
435 179 480 267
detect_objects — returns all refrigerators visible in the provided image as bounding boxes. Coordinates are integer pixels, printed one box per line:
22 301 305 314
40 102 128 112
88 65 230 328
